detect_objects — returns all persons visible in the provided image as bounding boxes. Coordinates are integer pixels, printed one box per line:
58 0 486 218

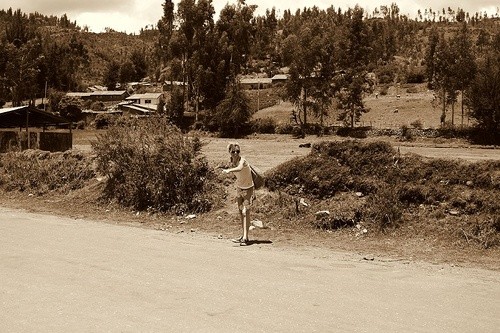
220 143 255 247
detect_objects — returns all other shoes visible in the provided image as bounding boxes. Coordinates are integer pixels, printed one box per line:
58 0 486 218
240 238 250 246
235 236 243 242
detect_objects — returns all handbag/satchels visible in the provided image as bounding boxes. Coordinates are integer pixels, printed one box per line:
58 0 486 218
251 171 263 189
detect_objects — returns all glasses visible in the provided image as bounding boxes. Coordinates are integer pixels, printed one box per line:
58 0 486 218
230 150 240 154
